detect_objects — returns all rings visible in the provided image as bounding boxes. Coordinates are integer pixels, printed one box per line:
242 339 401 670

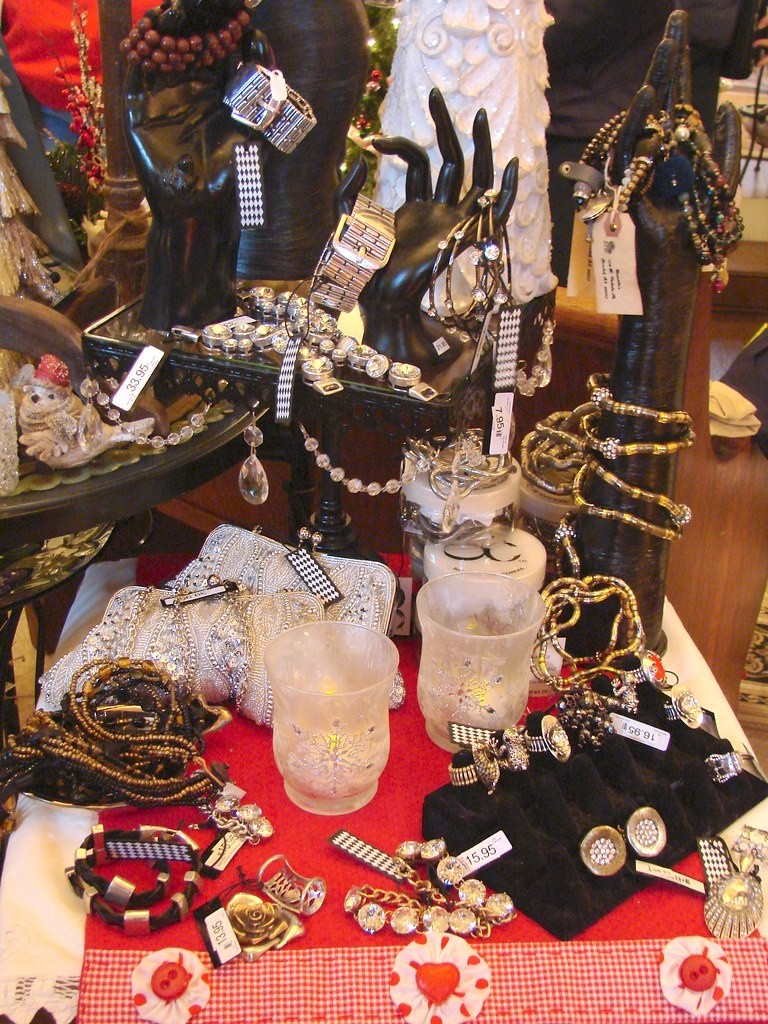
447 649 743 878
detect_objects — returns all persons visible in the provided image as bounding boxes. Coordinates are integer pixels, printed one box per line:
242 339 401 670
547 9 740 673
120 0 280 332
335 88 518 385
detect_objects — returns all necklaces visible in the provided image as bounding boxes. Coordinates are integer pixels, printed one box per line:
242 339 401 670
0 658 233 818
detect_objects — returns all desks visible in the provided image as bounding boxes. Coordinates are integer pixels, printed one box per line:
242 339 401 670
1 296 493 736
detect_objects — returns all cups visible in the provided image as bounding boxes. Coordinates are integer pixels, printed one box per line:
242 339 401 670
268 625 398 816
418 573 544 755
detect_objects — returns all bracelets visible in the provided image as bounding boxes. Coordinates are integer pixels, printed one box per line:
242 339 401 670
63 798 517 939
65 0 766 694
